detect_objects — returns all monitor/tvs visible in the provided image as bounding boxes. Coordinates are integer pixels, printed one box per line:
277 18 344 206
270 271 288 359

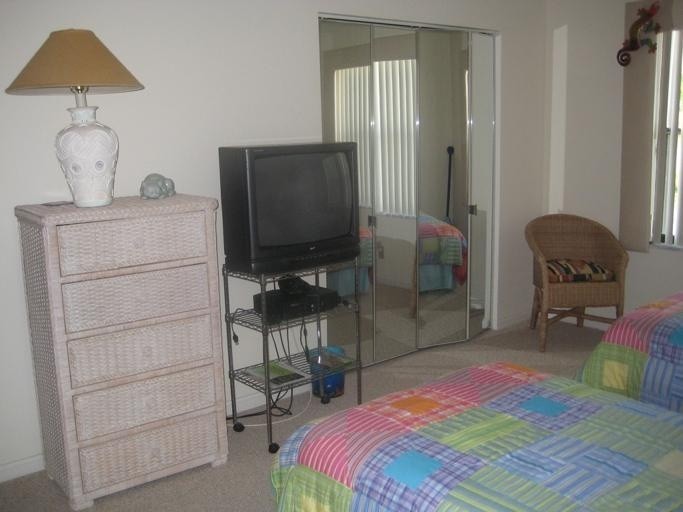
218 141 359 275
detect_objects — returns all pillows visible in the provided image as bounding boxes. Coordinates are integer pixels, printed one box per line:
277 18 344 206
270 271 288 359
547 257 614 283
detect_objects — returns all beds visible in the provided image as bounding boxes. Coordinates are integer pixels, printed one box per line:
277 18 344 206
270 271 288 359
328 205 467 295
271 363 682 511
581 288 683 411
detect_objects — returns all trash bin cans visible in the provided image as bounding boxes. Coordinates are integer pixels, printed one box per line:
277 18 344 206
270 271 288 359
309 347 345 397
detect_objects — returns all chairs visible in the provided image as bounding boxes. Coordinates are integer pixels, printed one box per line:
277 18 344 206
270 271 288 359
526 213 630 350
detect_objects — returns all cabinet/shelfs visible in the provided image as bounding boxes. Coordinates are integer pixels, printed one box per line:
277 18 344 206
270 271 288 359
222 257 362 453
13 194 228 512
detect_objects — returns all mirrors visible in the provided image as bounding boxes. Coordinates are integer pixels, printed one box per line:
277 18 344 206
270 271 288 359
318 17 495 372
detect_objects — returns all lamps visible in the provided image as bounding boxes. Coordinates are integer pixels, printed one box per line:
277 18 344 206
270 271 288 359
6 31 146 207
